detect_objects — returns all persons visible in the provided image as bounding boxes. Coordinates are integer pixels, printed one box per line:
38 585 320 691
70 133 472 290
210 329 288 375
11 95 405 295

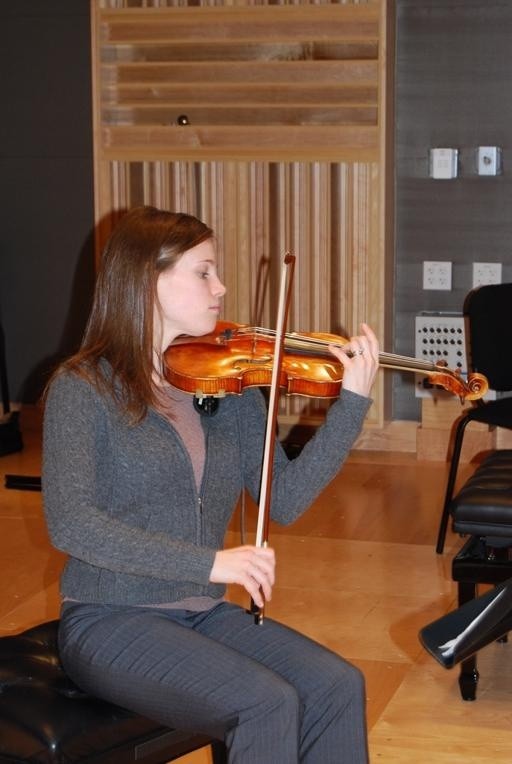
37 207 383 763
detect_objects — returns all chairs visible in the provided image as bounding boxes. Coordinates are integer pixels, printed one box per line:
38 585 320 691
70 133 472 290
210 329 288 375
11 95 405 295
436 281 512 554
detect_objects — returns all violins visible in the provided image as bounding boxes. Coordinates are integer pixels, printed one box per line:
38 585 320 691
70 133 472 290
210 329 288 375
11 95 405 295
163 320 488 404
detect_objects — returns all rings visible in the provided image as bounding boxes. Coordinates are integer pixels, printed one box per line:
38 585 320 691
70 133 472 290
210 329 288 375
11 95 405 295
351 349 364 357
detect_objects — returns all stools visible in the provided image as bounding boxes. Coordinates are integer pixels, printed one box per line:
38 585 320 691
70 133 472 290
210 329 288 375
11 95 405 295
1 620 228 764
